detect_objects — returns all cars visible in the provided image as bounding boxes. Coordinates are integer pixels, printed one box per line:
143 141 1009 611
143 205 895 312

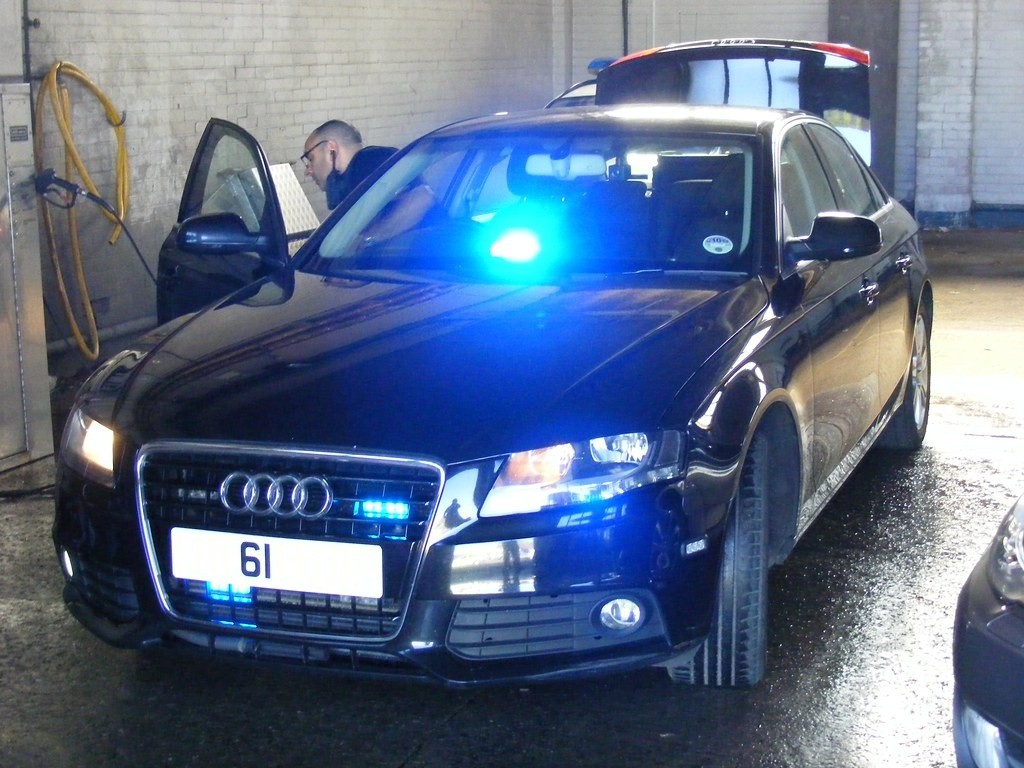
951 495 1024 768
51 37 935 696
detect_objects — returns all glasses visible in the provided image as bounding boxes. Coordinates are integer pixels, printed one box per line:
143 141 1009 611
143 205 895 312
299 140 331 168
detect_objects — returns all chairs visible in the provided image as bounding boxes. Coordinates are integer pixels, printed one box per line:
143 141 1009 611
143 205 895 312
577 179 650 264
660 181 742 273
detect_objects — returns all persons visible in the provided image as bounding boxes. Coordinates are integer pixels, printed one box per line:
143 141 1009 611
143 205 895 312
304 119 455 273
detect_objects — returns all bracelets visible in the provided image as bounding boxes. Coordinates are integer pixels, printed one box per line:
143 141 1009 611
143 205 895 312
362 231 374 247
